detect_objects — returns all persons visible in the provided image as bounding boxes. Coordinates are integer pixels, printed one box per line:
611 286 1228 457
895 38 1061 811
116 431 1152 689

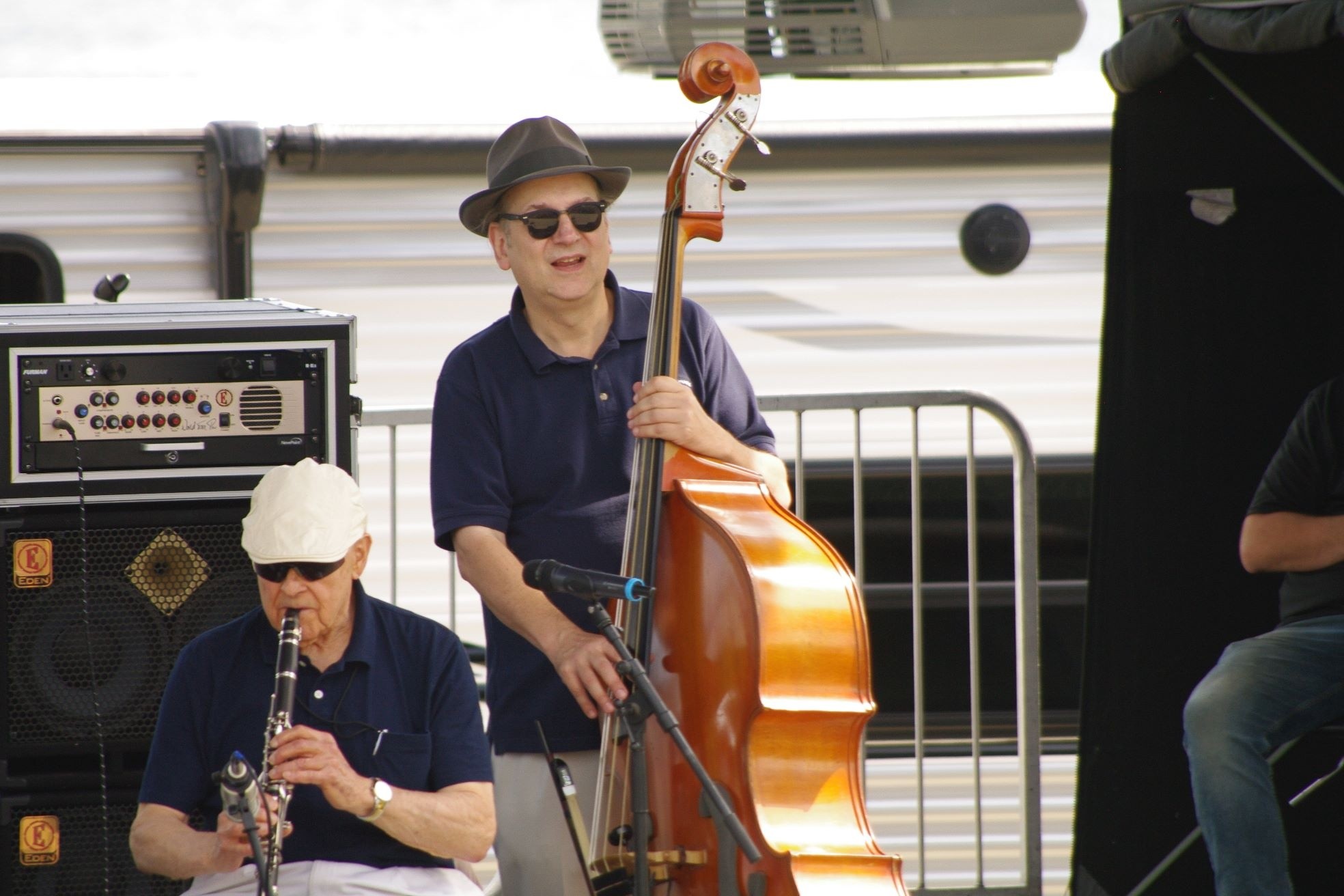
430 133 793 896
125 455 500 896
1183 375 1344 896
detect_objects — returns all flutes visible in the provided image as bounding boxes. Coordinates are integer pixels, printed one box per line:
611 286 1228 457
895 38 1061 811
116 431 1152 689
257 609 300 896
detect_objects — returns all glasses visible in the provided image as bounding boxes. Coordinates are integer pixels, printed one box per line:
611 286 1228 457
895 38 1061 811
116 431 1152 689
499 201 607 239
254 557 345 582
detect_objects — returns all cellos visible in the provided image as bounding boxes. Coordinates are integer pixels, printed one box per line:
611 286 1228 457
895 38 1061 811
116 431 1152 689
586 43 913 896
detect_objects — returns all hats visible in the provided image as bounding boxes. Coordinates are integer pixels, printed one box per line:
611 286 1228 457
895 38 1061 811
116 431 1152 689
241 457 372 564
458 116 632 239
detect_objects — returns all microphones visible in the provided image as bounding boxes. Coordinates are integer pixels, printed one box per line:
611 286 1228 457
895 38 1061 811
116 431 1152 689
219 747 261 822
522 559 656 599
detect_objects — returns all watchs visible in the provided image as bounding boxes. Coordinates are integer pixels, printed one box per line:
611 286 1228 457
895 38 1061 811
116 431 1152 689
358 776 393 822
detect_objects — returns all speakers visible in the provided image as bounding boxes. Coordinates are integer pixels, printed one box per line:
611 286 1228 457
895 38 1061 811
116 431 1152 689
1 507 260 786
1067 36 1343 896
0 791 226 896
